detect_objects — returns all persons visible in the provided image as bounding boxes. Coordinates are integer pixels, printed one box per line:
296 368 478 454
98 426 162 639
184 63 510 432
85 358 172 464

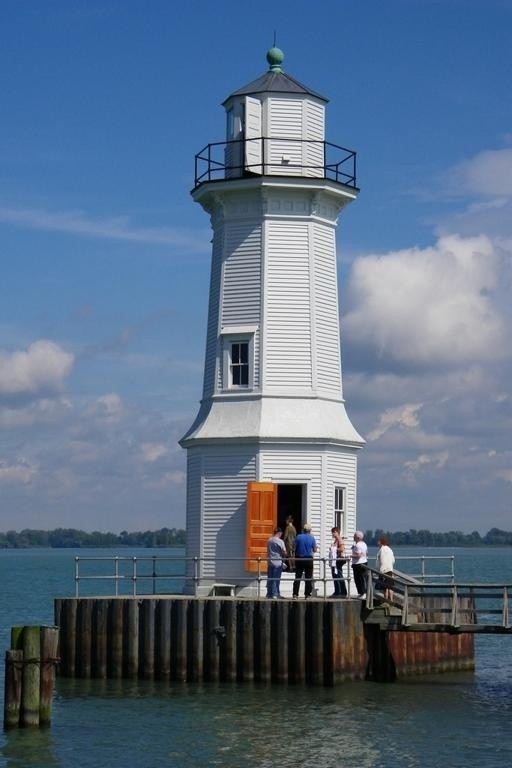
349 531 368 599
266 528 288 598
328 526 347 598
292 524 317 600
283 515 297 572
375 537 395 608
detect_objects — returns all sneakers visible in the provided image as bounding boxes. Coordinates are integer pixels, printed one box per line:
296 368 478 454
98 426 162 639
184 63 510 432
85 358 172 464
265 592 395 609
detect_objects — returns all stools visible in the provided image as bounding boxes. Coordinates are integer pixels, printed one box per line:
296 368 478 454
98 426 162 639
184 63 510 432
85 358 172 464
211 583 238 596
